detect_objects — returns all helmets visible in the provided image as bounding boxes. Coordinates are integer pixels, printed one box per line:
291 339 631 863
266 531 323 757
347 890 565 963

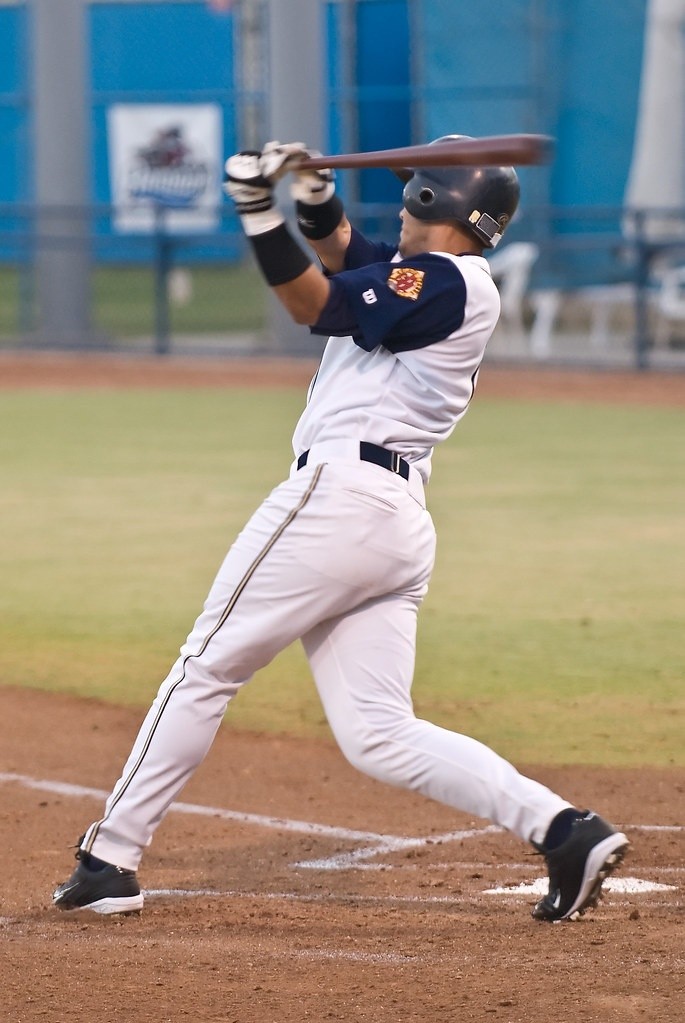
396 135 521 248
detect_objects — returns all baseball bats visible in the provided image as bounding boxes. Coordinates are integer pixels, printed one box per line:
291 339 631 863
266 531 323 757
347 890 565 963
286 134 554 172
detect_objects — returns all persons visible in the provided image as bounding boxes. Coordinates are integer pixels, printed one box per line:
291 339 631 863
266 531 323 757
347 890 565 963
52 135 630 923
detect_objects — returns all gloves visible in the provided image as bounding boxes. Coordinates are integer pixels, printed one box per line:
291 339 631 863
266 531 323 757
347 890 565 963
224 150 285 237
261 141 335 203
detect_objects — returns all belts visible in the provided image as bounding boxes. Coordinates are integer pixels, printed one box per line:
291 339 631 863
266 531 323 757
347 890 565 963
297 441 409 482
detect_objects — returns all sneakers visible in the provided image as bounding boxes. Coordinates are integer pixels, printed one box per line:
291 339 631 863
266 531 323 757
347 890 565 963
53 861 144 915
530 810 634 924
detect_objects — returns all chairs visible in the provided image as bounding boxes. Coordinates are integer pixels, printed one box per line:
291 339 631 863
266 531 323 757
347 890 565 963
482 242 685 362
